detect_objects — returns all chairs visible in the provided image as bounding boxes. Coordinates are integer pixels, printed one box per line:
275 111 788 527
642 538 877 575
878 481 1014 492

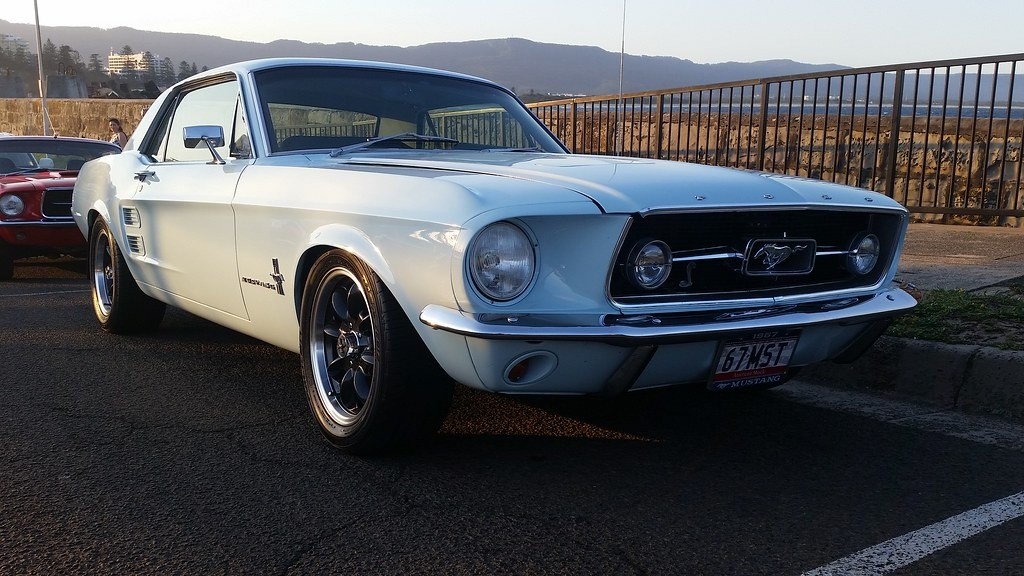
67 159 88 170
0 158 19 175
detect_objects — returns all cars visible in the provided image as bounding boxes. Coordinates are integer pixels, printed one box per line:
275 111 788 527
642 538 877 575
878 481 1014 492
70 55 920 449
0 132 122 281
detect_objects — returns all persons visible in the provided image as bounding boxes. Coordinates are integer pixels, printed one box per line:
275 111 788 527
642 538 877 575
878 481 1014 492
107 119 128 150
